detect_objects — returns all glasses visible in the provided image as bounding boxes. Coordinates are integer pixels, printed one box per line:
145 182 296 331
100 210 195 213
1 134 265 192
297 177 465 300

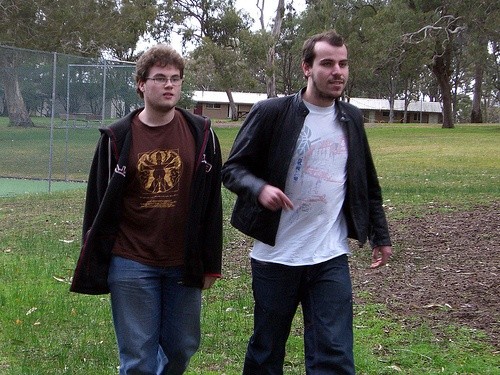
141 77 184 86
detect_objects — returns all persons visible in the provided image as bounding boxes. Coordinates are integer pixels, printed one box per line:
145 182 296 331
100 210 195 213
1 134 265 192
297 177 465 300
221 30 393 375
68 46 222 375
